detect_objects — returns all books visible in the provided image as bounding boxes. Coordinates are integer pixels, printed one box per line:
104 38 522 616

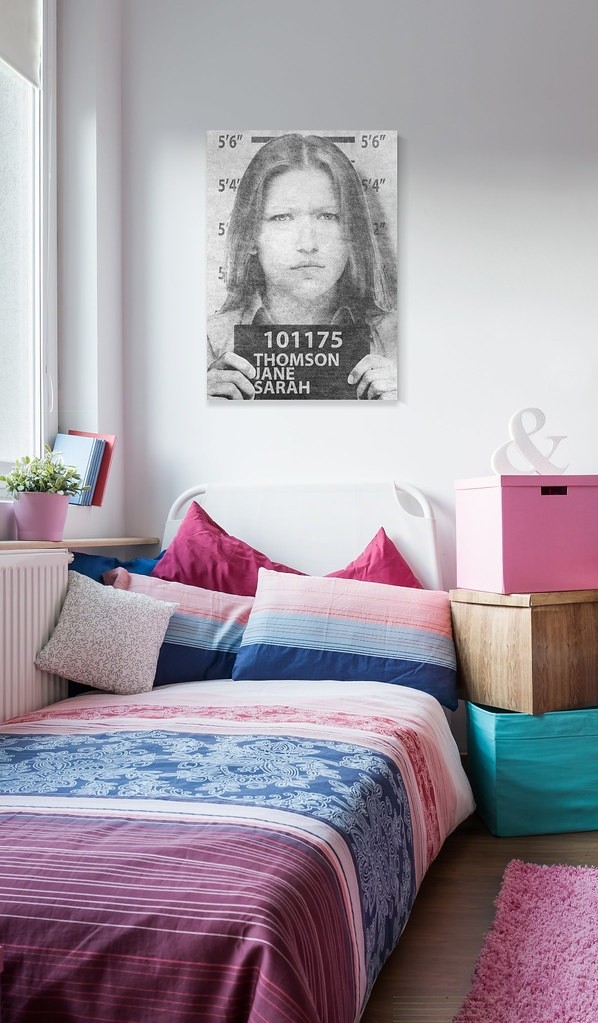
52 430 118 506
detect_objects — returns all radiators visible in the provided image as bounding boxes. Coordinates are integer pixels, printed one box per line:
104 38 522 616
0 548 68 724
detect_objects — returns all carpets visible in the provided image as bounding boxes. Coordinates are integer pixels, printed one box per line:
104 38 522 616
453 858 598 1023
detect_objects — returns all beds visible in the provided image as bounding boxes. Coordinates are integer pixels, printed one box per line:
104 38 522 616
0 477 477 1023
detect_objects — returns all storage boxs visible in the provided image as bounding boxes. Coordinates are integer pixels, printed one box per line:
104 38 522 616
466 701 598 838
454 474 598 595
447 589 598 717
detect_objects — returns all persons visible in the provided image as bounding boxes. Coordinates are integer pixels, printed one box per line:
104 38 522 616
207 133 399 401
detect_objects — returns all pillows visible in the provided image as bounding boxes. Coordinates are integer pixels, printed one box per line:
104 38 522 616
34 570 179 695
233 567 459 712
151 500 310 597
69 549 167 586
320 526 425 591
102 567 255 687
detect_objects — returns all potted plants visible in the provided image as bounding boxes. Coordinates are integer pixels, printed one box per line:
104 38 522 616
0 444 91 542
